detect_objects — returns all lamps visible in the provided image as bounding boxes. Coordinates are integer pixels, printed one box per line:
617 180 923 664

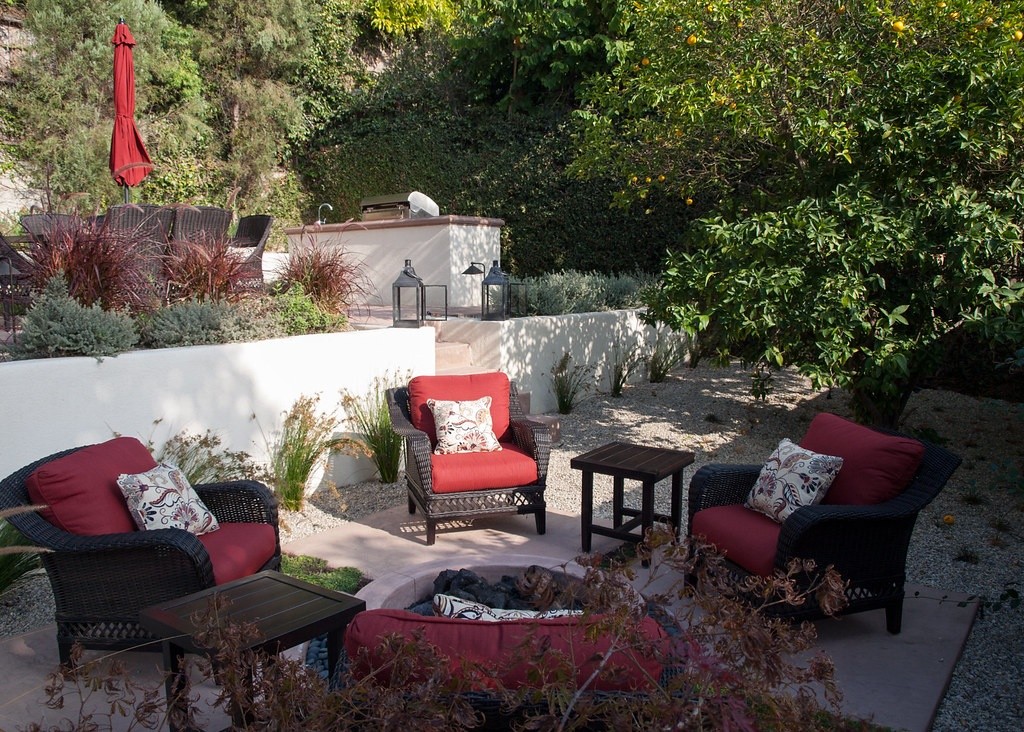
391 260 449 328
462 261 528 320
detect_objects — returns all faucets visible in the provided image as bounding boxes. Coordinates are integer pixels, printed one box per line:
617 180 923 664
318 203 333 225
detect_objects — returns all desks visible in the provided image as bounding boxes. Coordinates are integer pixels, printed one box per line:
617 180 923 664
570 442 695 568
138 568 367 732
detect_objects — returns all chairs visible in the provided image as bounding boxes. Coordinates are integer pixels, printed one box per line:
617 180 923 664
346 595 685 732
388 373 553 545
681 413 960 634
0 204 273 331
0 438 281 675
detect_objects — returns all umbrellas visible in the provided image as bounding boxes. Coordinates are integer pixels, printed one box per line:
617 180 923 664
110 23 154 207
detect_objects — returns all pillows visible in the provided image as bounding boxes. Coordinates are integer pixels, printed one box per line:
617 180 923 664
118 460 221 537
745 437 842 524
425 395 503 455
431 594 586 622
